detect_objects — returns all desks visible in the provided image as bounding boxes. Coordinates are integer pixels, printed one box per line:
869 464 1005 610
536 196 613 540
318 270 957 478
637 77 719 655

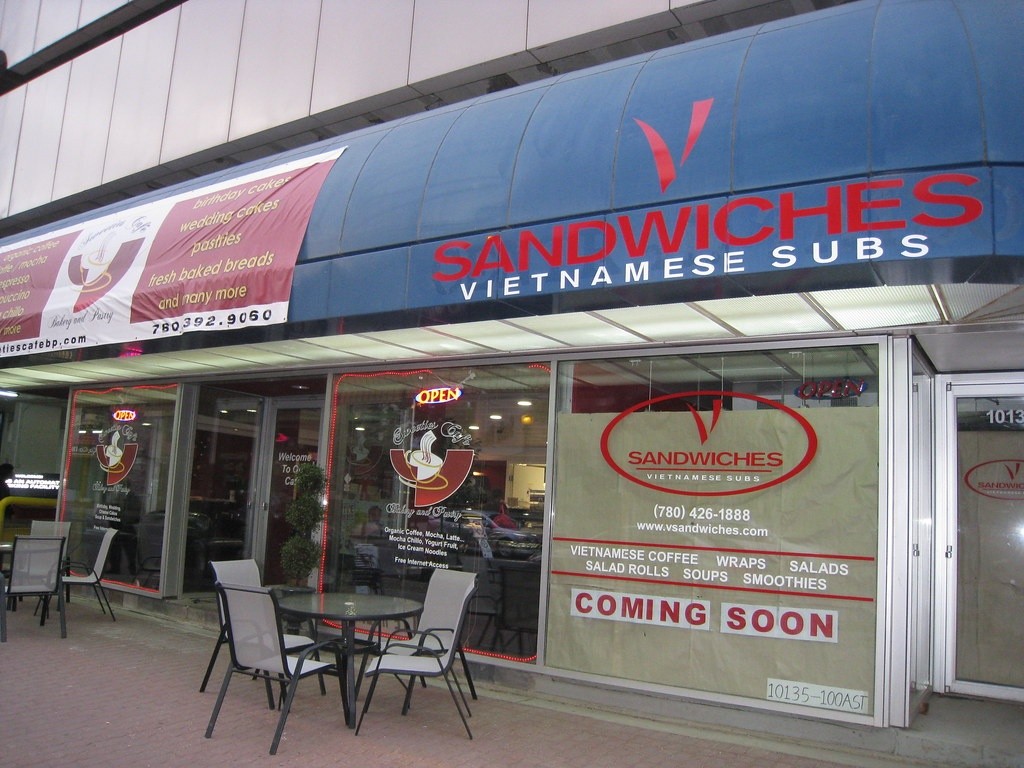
281 591 424 728
1 546 72 613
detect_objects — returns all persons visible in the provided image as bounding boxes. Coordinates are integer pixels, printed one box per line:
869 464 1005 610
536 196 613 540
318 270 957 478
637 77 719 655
104 479 148 587
486 487 516 529
352 506 383 537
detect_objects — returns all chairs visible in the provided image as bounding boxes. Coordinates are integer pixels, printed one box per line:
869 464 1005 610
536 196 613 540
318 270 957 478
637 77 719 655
458 554 542 665
199 560 478 756
0 518 120 642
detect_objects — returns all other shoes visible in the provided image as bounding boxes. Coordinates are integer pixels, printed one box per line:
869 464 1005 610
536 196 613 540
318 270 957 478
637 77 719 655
106 570 120 575
